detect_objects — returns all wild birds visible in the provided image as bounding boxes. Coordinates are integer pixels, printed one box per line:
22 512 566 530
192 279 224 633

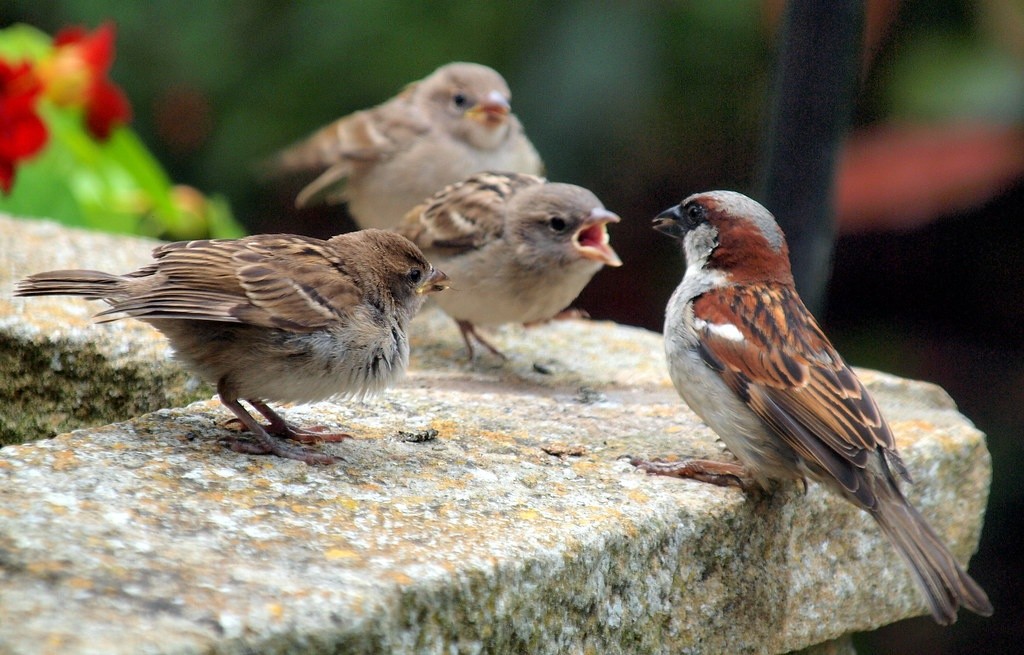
392 170 623 370
616 191 994 625
11 229 451 468
271 62 544 229
